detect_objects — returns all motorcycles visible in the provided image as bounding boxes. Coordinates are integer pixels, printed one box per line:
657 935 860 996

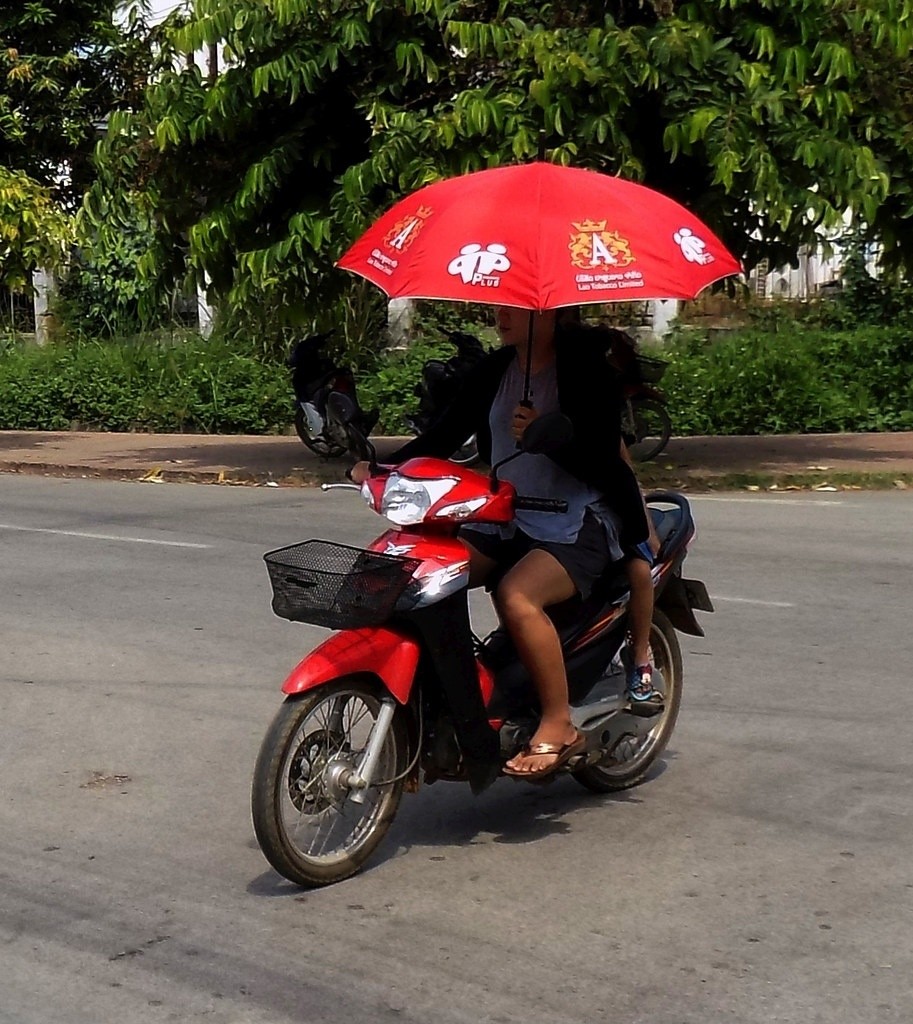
605 324 673 464
247 385 718 890
400 323 491 466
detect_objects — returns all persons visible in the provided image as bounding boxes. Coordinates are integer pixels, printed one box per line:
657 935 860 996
351 306 650 778
620 435 661 702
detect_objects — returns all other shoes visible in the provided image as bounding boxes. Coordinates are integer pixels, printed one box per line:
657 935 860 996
448 442 480 463
627 665 663 705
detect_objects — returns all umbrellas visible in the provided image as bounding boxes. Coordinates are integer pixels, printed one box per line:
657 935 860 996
336 128 744 451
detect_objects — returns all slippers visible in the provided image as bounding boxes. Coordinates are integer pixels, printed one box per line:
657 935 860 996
502 731 586 776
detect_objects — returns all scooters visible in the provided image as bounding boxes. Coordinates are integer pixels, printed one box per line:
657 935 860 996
279 326 380 462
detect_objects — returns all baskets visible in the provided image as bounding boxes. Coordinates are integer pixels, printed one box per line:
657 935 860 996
635 355 666 384
265 541 423 629
422 359 447 381
